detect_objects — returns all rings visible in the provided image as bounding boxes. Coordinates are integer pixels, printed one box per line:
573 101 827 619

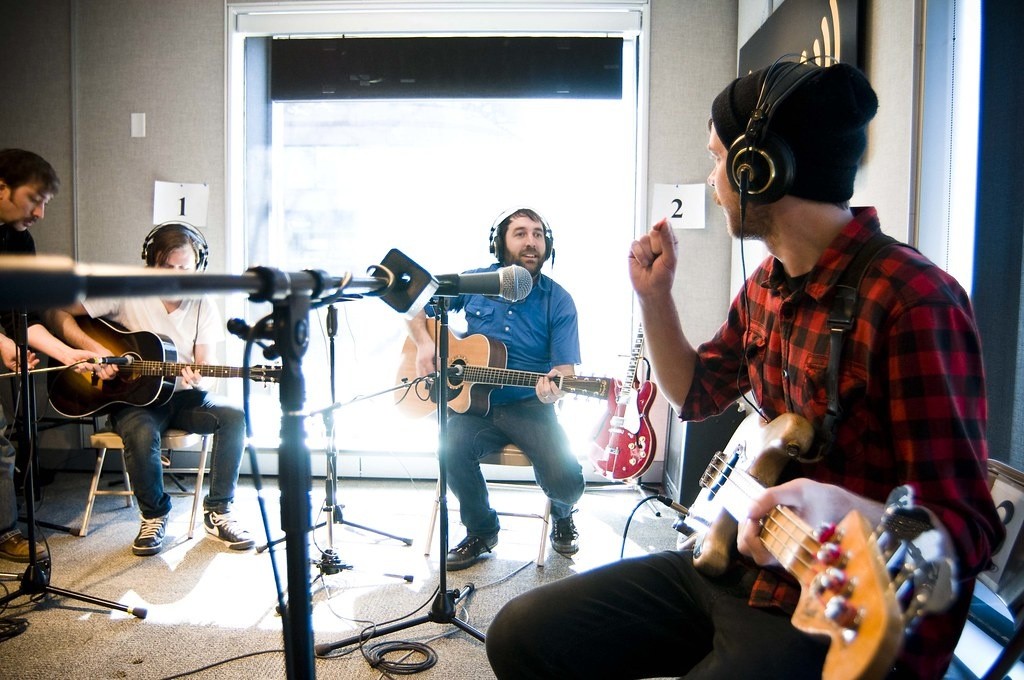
542 395 549 399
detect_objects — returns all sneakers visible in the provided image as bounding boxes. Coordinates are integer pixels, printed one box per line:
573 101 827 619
204 509 255 549
447 533 498 570
132 511 169 555
550 505 581 553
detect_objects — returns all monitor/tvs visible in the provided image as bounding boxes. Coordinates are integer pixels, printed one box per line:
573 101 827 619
662 401 747 508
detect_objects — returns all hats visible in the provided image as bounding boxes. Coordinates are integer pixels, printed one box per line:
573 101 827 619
711 60 880 203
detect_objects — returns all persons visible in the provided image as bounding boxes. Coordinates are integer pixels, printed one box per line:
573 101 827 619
405 205 586 572
485 64 1007 680
0 148 109 563
40 221 255 556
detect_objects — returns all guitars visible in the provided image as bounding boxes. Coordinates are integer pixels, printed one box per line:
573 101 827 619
587 321 658 483
394 317 611 422
676 410 961 680
44 312 283 418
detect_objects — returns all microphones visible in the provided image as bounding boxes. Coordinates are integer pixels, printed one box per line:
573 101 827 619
87 354 134 365
432 265 534 302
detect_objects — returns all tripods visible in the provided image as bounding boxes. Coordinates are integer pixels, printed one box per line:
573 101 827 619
582 355 662 516
0 309 148 620
253 287 490 659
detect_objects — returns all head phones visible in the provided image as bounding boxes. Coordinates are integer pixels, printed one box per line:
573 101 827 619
489 209 553 264
141 220 209 273
726 52 839 206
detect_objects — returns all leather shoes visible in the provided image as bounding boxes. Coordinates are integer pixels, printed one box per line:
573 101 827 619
0 529 46 561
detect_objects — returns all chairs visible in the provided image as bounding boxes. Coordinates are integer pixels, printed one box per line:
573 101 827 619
423 441 553 568
80 425 211 543
